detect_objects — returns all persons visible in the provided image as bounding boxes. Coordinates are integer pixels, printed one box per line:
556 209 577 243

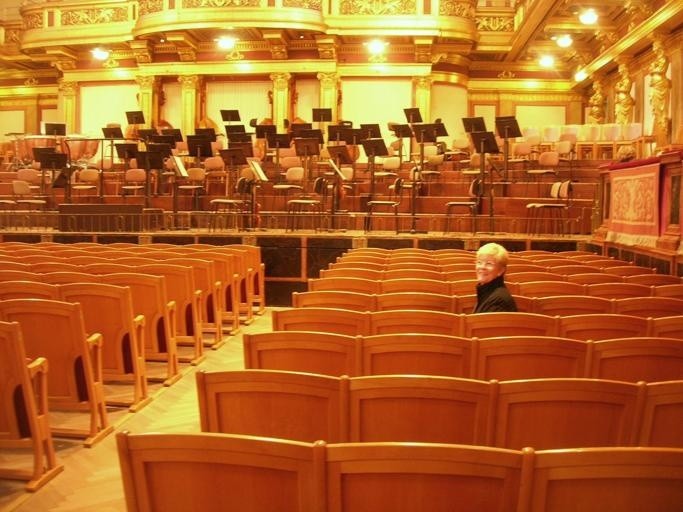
470 242 517 315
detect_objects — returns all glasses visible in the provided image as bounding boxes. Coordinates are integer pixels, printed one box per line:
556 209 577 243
475 260 502 266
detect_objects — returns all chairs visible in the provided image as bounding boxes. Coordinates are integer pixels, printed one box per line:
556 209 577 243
0 122 680 511
